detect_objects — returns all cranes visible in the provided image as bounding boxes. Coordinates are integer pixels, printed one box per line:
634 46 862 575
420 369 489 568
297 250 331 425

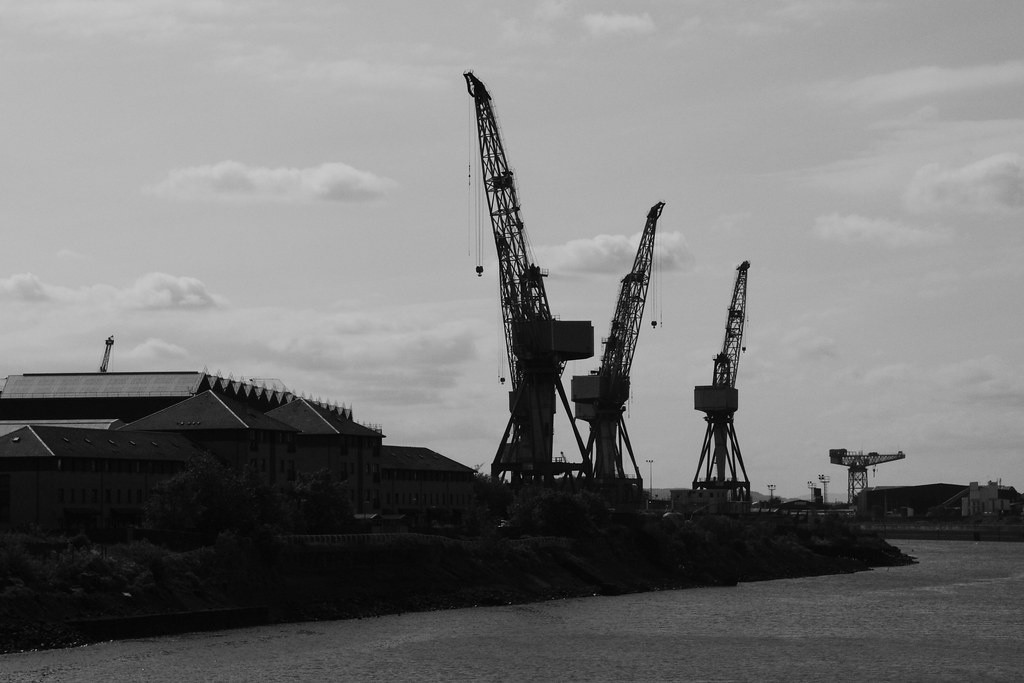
828 447 906 519
569 199 668 517
689 260 753 519
462 67 598 499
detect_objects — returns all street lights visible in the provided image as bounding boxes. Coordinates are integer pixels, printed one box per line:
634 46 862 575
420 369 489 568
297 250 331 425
645 459 654 498
767 484 777 511
818 474 830 506
